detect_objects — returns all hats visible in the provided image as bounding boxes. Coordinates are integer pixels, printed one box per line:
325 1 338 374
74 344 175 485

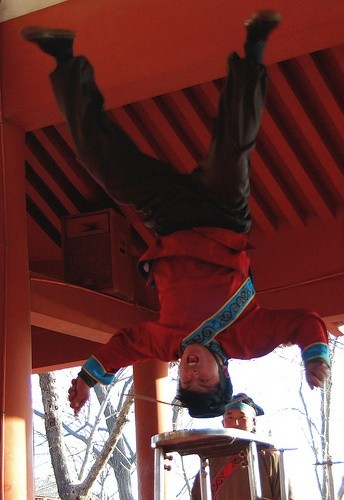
223 392 264 417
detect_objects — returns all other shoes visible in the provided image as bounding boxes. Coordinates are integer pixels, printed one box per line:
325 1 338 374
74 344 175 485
243 10 281 37
22 28 75 55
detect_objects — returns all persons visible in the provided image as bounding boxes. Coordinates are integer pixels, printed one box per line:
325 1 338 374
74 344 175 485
189 393 293 500
20 9 332 414
